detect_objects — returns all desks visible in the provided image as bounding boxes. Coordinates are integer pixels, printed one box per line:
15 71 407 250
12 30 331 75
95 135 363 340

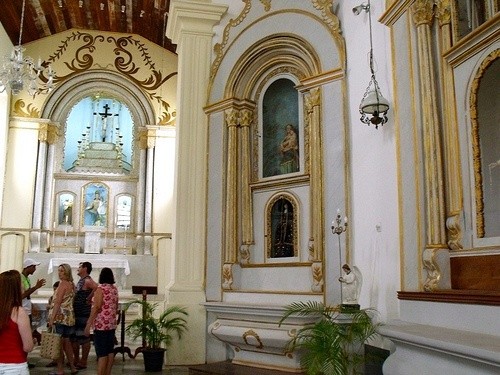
30 302 133 361
47 259 130 288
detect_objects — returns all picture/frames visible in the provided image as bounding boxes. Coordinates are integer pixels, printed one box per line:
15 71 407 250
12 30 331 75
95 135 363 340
80 182 110 228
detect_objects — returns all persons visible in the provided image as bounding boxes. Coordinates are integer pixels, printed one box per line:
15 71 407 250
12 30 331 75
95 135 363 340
20 258 46 368
86 191 106 226
279 124 298 174
0 269 34 375
84 267 119 375
45 281 65 367
47 264 79 375
66 261 97 370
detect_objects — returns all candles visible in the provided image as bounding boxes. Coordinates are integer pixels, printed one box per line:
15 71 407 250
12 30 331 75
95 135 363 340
53 222 55 228
114 217 117 224
66 216 68 222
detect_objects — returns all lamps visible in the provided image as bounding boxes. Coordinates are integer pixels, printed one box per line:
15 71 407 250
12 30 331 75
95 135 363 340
353 0 389 129
121 6 125 13
58 0 62 7
79 1 83 7
100 3 104 10
0 0 52 95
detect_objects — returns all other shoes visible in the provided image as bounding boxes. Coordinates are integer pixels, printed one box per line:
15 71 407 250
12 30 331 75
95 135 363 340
28 364 36 369
45 361 58 367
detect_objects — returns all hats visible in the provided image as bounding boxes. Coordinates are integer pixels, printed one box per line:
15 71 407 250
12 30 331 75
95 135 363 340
23 258 41 269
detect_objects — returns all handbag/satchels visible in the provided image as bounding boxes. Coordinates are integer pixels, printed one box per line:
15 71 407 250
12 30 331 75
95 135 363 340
40 326 61 360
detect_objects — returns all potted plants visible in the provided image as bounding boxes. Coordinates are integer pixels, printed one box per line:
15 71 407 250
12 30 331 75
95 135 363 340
127 299 188 373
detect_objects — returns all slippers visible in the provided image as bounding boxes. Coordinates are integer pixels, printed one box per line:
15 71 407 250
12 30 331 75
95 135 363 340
68 371 79 375
65 362 80 367
71 365 86 370
48 371 59 375
